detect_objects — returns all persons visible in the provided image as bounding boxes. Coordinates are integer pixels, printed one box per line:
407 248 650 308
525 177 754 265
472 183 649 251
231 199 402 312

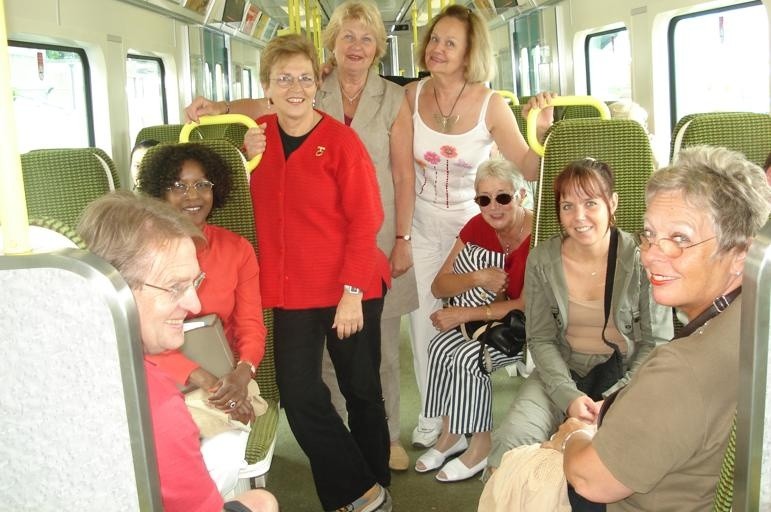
480 157 677 488
415 160 534 484
313 6 559 446
237 34 392 512
139 142 267 501
476 143 771 512
129 138 160 191
184 0 414 472
73 189 279 512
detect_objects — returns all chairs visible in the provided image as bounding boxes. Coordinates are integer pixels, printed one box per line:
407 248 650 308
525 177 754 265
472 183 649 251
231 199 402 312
0 122 286 512
507 96 771 512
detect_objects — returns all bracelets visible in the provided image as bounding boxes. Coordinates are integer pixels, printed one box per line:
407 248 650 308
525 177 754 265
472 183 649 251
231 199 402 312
223 100 230 114
561 428 592 452
237 360 256 379
481 305 491 323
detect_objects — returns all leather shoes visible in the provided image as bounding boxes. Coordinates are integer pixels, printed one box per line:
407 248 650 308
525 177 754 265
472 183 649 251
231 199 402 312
386 438 409 471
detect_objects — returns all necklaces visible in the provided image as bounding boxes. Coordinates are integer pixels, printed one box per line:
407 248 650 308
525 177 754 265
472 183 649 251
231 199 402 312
494 207 526 255
591 270 597 276
430 76 469 130
337 77 367 107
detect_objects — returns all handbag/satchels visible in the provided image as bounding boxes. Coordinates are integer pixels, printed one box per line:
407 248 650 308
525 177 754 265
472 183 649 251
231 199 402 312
571 346 625 403
448 300 525 374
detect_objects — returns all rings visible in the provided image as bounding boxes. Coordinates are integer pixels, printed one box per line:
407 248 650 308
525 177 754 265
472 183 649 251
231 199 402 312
228 399 236 409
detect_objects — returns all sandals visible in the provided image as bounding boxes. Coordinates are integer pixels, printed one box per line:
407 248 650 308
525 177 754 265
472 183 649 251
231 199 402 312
436 447 488 482
414 432 468 473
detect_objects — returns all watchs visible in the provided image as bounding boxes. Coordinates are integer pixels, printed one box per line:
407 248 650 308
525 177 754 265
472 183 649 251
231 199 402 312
395 235 412 241
342 283 362 296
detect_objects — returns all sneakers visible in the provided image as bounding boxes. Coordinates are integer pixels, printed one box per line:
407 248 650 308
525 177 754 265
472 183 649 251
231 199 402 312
412 412 443 448
332 483 386 512
361 484 392 511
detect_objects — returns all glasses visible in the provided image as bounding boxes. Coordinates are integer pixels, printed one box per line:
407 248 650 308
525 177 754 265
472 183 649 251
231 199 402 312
271 73 315 88
165 179 213 195
474 193 516 206
142 273 206 301
633 231 719 258
